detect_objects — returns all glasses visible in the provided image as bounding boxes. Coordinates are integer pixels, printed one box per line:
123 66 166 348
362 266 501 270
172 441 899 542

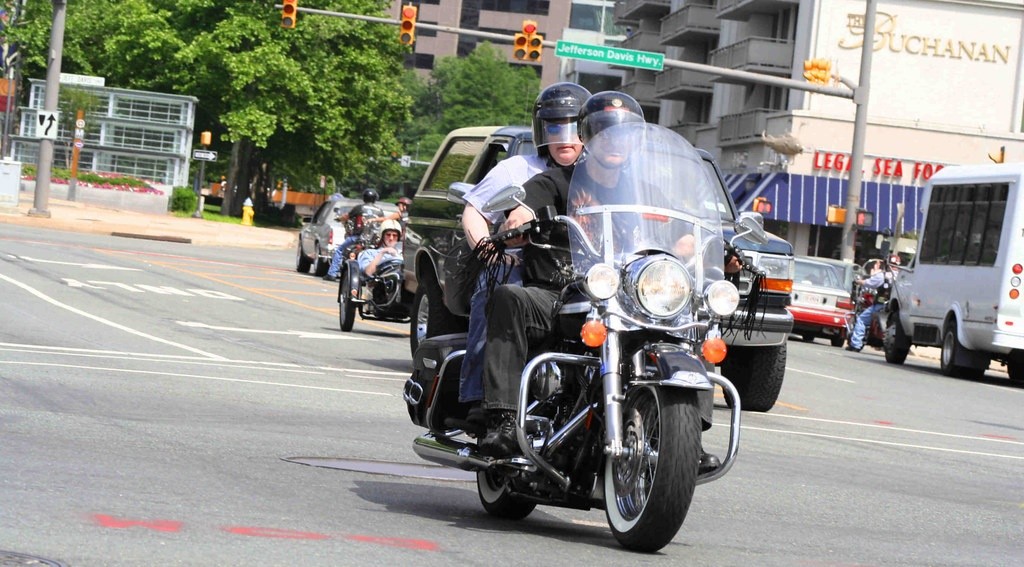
544 124 578 135
386 233 398 237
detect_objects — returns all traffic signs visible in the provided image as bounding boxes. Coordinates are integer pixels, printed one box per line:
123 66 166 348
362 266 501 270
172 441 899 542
193 148 218 162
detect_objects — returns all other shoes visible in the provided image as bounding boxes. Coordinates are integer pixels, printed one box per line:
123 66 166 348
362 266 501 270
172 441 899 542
323 274 336 281
846 345 860 352
465 405 485 438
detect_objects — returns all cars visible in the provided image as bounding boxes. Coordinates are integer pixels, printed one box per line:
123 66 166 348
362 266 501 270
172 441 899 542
294 196 400 276
787 254 892 350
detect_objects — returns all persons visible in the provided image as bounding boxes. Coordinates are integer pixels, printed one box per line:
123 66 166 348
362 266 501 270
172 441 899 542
845 254 901 353
808 275 817 284
458 80 744 474
323 188 413 281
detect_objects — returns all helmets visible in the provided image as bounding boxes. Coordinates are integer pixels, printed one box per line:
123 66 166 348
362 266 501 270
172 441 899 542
396 197 413 206
887 254 901 264
363 188 378 201
381 219 402 240
532 82 591 143
577 91 644 142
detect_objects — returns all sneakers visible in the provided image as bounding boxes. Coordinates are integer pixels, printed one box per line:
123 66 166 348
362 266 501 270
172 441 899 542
479 412 523 457
698 448 720 473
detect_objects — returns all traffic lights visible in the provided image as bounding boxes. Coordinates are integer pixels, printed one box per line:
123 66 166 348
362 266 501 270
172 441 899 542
756 201 772 214
855 210 874 228
802 58 832 85
281 0 298 29
988 145 1007 163
513 19 543 62
399 5 417 45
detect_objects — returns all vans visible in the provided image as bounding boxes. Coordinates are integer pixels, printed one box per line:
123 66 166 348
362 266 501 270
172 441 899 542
877 161 1024 385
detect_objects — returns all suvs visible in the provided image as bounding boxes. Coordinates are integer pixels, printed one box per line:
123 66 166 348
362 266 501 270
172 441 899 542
398 126 796 414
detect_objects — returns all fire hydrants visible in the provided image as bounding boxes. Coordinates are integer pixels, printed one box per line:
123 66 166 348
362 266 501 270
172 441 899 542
240 198 256 226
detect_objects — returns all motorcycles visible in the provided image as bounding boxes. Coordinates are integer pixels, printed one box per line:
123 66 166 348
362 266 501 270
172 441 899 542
329 207 412 332
405 121 769 554
